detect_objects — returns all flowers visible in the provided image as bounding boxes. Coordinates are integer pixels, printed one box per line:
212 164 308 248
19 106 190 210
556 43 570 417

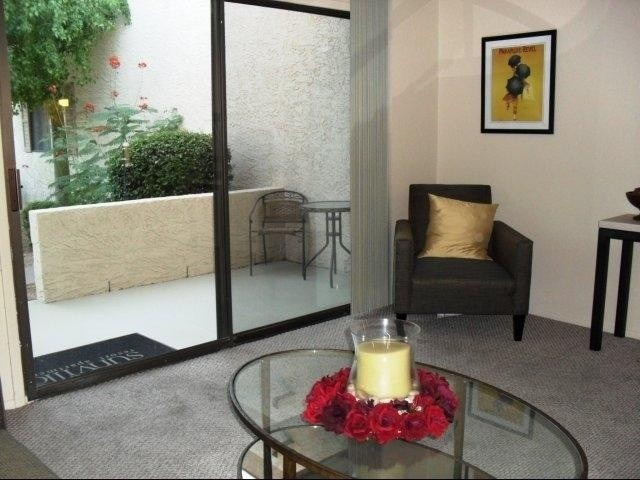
301 367 460 444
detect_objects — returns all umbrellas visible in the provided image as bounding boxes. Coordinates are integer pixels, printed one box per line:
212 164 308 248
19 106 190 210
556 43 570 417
506 54 531 96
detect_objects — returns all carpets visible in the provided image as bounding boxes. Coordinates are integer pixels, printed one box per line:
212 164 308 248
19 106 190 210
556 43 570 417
1 302 640 480
30 333 177 394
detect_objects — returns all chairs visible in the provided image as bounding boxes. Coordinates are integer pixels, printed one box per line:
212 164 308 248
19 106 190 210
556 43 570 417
249 191 309 280
392 184 534 341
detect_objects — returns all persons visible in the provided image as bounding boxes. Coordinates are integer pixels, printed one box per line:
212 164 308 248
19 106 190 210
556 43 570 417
503 91 518 120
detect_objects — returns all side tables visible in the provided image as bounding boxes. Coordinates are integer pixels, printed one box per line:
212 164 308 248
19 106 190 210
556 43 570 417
300 201 352 287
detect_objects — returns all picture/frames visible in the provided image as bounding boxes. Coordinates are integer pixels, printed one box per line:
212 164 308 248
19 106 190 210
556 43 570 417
467 379 534 440
481 30 556 134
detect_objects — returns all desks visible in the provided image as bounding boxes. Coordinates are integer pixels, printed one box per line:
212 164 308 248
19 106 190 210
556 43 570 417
589 214 640 351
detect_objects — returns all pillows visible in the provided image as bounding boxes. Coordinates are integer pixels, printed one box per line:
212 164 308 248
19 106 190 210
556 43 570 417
416 192 499 261
